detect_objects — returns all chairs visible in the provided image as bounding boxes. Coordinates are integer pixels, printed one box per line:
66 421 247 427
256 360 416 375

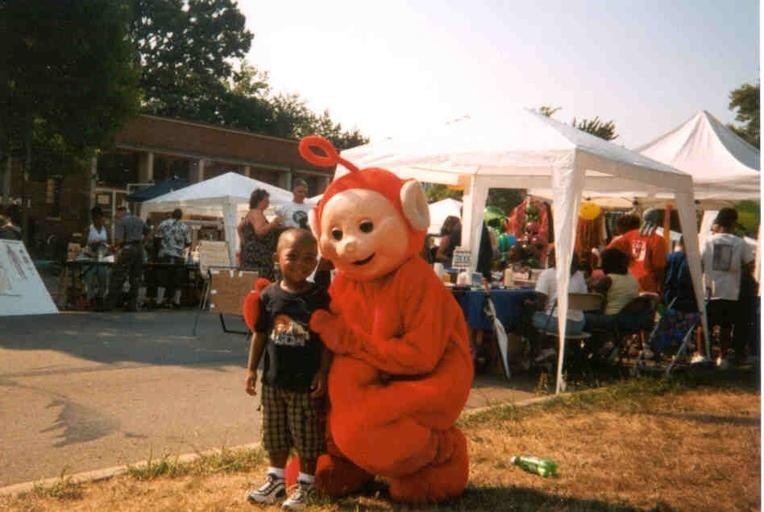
539 293 602 386
608 292 658 375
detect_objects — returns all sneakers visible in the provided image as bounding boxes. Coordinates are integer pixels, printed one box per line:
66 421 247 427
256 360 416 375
535 346 558 363
282 480 316 512
690 352 729 368
246 472 285 505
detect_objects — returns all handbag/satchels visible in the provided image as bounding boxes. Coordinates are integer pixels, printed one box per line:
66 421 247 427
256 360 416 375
511 308 536 337
667 285 698 313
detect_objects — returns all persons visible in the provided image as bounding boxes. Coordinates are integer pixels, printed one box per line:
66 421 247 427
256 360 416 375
237 177 335 510
105 205 149 313
436 208 462 270
476 194 589 374
82 207 112 303
423 234 436 263
145 208 193 310
584 206 761 368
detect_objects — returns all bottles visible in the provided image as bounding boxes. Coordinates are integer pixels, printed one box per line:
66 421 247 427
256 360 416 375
448 267 466 283
451 245 472 268
510 454 557 477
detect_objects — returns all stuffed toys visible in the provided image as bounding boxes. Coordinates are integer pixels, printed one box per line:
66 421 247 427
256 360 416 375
245 137 476 503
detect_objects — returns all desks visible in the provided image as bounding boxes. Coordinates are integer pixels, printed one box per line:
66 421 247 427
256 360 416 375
452 280 537 381
55 258 199 311
